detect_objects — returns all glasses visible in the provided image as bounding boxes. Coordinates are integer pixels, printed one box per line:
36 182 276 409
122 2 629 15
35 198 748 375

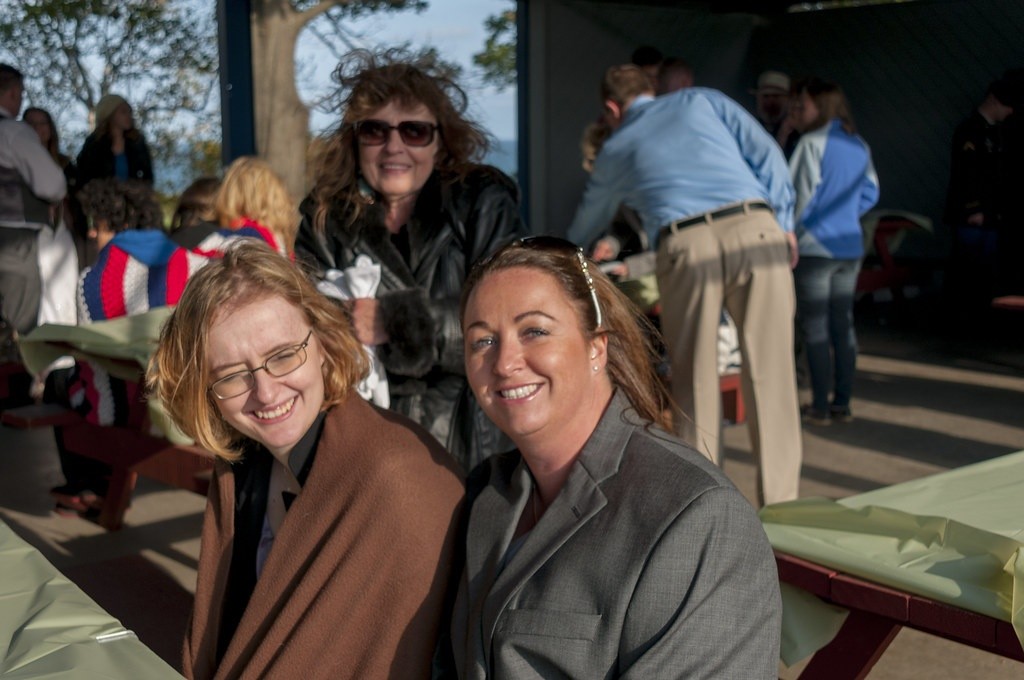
206 328 311 400
353 118 439 146
475 237 601 328
87 215 109 227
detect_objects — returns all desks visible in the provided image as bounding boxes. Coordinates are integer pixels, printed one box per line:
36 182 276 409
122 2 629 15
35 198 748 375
758 451 1023 679
14 305 215 531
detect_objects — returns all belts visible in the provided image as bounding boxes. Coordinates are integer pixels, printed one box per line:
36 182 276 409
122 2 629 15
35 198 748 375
653 202 771 251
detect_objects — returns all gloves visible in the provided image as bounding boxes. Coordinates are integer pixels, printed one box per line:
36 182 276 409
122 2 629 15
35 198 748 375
319 254 389 409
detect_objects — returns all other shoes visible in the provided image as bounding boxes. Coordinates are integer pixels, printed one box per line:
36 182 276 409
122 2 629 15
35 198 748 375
800 405 832 427
831 407 853 422
49 482 109 518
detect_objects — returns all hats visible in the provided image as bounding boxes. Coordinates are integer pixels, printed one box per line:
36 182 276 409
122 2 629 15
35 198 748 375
757 71 789 92
96 95 124 124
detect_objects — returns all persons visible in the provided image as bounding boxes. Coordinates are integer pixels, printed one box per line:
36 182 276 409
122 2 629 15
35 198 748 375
165 158 292 308
292 48 530 477
144 243 474 679
0 64 68 428
448 234 784 680
787 81 879 428
565 64 802 506
166 177 222 249
658 59 693 94
77 94 154 269
950 81 1016 352
21 107 87 250
741 70 790 140
48 174 211 520
631 46 662 96
